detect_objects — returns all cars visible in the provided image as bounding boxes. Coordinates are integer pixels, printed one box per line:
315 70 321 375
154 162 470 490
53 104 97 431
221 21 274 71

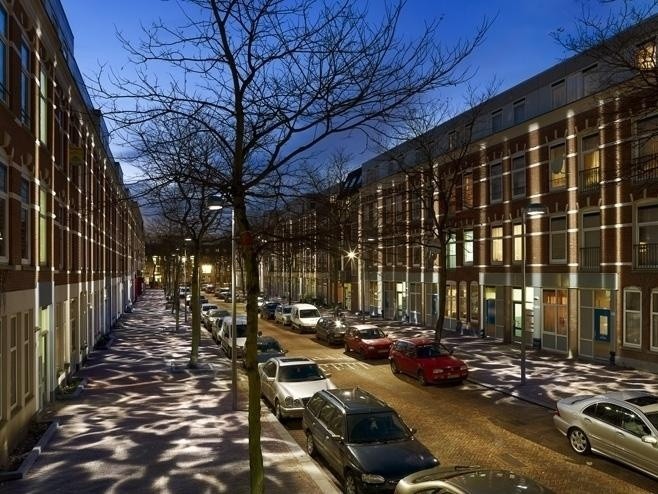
258 297 292 325
344 325 392 358
256 356 339 421
554 390 658 480
302 387 440 494
390 336 469 386
224 289 241 303
394 465 557 494
179 284 232 344
317 316 349 344
244 336 288 368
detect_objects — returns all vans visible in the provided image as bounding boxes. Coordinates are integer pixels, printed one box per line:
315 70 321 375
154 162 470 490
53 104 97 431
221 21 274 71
220 316 248 359
290 304 322 332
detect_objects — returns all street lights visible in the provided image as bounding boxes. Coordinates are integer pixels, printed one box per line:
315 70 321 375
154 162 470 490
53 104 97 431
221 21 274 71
185 238 201 340
519 204 547 384
206 202 238 411
346 250 366 323
